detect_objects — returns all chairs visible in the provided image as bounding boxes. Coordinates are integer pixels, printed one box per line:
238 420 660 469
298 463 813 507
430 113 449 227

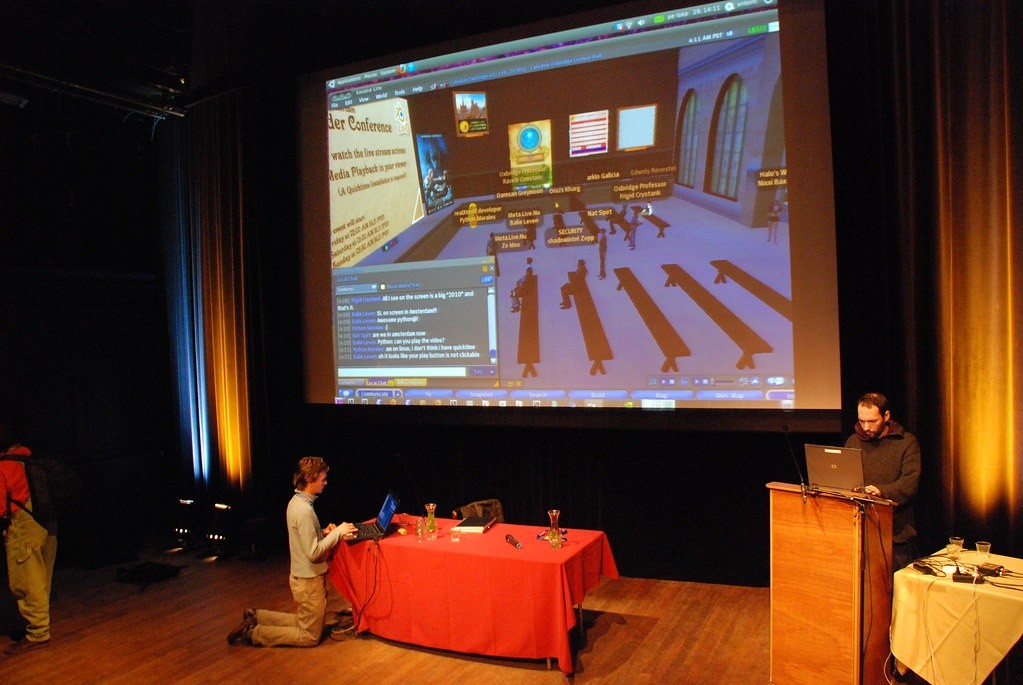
452 498 504 523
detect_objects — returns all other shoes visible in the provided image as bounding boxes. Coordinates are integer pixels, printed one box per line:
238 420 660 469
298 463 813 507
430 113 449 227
227 618 252 643
244 608 255 620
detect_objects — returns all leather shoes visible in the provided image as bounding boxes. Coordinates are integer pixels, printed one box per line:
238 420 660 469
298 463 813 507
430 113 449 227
6 635 49 653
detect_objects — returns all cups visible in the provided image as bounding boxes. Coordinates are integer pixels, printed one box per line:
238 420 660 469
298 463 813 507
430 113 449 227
949 537 964 552
946 544 959 560
450 528 460 542
399 513 409 527
976 541 991 559
415 518 426 541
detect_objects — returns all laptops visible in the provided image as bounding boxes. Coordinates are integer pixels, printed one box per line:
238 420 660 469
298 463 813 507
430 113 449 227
343 490 401 541
804 444 865 490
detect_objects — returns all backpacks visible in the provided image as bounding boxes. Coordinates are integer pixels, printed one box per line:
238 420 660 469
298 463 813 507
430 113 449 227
0 447 72 523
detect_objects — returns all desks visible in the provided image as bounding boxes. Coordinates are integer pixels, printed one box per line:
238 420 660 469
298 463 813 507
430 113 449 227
322 514 620 676
888 544 1023 685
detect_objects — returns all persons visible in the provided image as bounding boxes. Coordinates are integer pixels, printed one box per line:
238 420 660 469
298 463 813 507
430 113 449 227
844 393 921 573
227 457 357 648
0 424 57 654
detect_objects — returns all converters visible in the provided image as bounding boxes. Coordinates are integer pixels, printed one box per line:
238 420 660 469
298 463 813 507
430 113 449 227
978 562 1004 577
913 563 933 574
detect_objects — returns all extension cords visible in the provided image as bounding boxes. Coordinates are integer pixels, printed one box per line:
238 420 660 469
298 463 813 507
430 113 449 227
952 573 983 584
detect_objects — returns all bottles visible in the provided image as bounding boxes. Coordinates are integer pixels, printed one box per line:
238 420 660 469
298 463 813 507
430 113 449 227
548 510 562 548
425 503 439 541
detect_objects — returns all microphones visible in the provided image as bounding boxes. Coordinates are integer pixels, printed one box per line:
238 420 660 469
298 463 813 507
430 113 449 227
506 535 522 548
